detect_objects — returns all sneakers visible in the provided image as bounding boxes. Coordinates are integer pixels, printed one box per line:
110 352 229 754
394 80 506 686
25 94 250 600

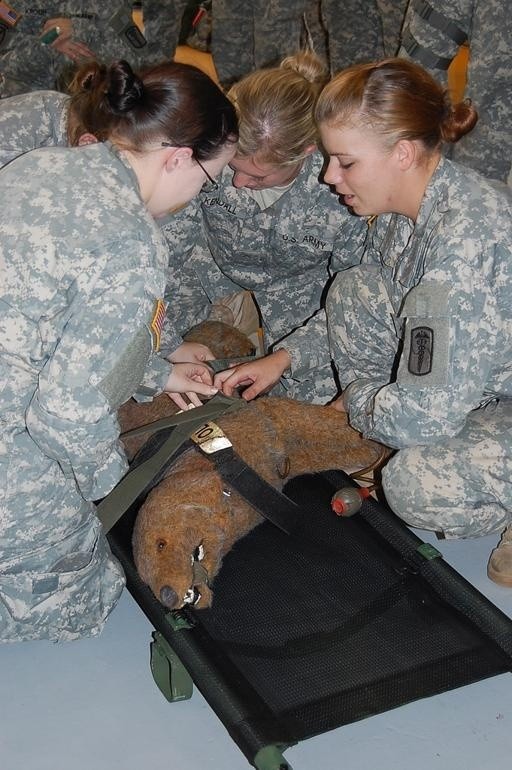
211 292 260 337
486 522 512 588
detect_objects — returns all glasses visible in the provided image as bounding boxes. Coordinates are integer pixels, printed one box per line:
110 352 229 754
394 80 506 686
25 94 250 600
161 141 219 193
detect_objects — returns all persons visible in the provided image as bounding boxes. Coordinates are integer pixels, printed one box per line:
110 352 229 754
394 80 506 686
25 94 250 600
1 1 511 641
